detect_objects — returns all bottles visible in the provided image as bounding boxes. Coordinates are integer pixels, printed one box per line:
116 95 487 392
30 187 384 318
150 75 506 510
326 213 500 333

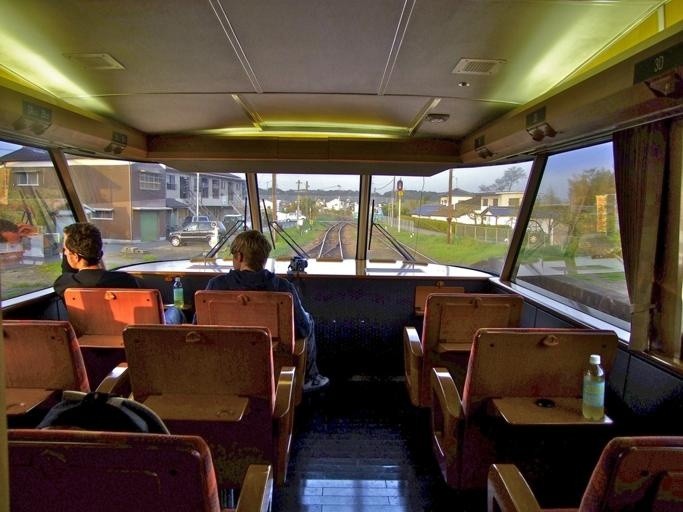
174 277 184 308
582 355 606 421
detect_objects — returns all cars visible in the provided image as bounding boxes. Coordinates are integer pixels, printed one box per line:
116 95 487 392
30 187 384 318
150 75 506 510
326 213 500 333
166 212 311 249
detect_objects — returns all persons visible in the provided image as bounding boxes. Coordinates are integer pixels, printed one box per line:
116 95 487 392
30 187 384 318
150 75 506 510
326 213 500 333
53 220 188 326
191 230 331 394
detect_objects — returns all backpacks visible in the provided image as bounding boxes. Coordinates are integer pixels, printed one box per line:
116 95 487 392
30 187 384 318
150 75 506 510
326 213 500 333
37 390 169 434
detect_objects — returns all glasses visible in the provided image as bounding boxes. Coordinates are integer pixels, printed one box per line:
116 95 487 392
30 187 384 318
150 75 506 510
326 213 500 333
77 251 103 263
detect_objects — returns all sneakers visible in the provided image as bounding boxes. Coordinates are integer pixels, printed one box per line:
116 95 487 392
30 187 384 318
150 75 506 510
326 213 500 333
303 374 329 392
